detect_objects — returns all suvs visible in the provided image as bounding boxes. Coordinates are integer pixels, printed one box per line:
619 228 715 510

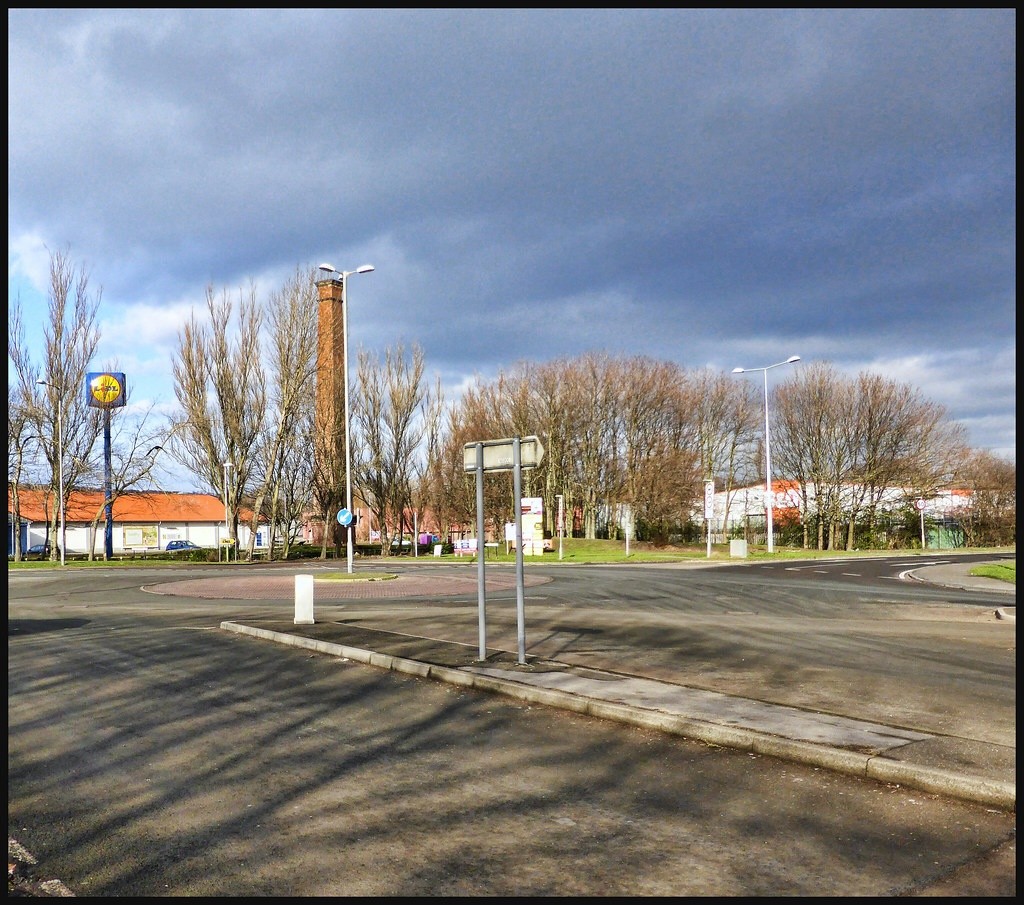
27 545 58 561
165 541 202 553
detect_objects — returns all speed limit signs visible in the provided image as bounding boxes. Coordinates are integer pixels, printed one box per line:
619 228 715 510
916 499 925 509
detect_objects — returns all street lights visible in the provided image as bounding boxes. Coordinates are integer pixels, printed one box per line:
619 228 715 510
733 354 802 552
36 379 66 567
320 261 375 573
222 463 235 561
553 495 563 559
703 479 711 559
